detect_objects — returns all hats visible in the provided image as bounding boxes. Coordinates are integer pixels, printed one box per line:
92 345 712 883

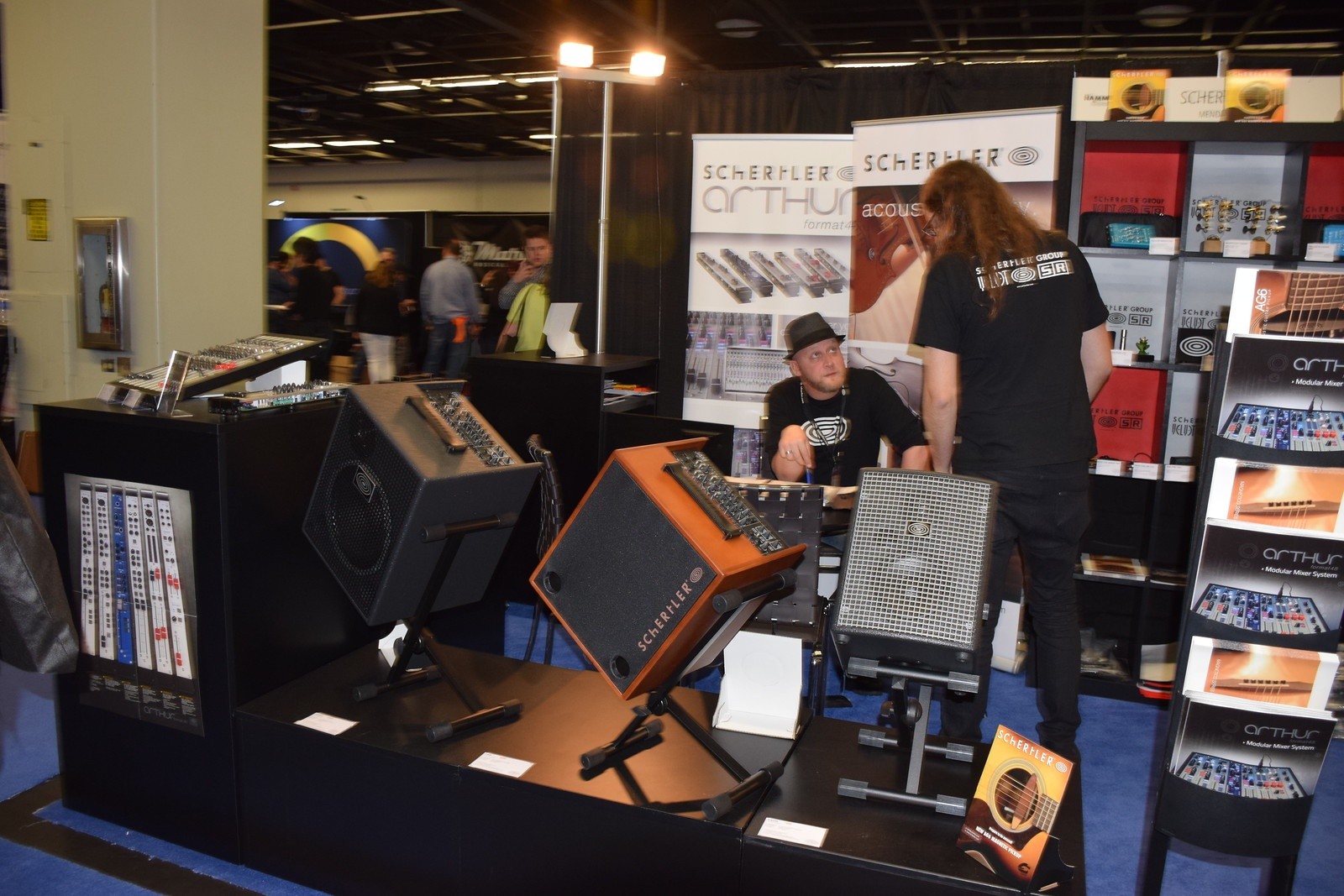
782 312 846 360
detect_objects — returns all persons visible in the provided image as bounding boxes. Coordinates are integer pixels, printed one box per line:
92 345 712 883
745 312 931 695
267 216 555 384
915 159 1113 756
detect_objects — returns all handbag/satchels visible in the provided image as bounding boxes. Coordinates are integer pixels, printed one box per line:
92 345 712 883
0 436 82 678
494 335 519 354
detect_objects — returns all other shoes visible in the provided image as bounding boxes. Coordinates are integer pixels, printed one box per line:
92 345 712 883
1062 745 1081 765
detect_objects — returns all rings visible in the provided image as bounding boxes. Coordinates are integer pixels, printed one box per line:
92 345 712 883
785 450 792 454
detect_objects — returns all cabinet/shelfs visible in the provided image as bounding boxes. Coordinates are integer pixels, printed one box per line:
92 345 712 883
1068 122 1344 710
465 348 734 605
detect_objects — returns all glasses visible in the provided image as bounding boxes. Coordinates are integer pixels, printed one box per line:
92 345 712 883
920 204 944 239
